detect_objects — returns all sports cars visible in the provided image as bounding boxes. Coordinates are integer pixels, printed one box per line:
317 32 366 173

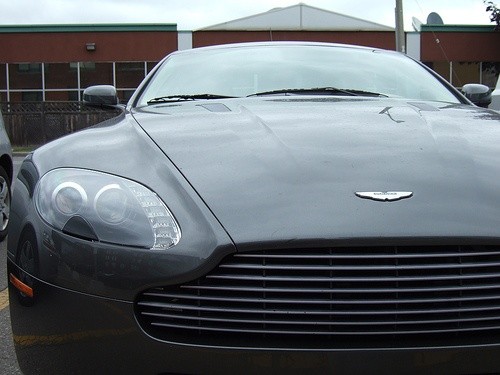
9 41 500 375
